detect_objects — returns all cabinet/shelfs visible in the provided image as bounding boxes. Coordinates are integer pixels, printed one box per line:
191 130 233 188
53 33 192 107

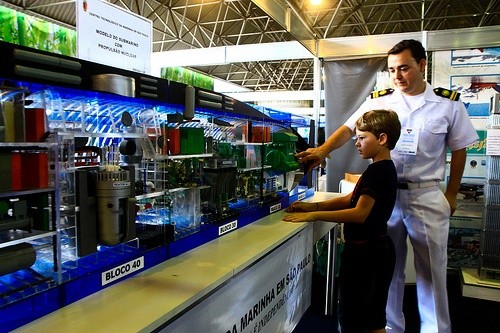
1 132 62 287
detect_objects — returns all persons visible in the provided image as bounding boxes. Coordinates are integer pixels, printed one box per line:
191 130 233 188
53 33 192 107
295 38 480 333
283 107 400 333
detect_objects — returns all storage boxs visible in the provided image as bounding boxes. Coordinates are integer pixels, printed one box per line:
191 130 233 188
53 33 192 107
147 126 205 157
1 101 48 193
478 95 500 282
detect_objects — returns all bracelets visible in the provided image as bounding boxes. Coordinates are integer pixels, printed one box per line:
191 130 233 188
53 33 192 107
316 202 319 210
